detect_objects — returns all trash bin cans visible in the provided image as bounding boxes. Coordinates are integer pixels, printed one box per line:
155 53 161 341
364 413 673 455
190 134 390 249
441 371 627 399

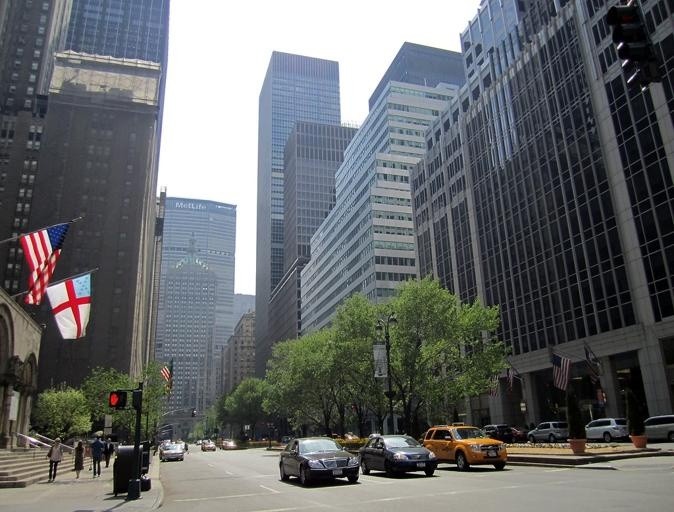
114 444 144 498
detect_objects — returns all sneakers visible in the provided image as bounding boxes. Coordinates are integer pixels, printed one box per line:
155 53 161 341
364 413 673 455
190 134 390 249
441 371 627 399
48 466 109 483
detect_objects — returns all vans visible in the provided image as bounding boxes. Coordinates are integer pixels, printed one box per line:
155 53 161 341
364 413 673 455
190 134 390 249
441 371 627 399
641 414 674 444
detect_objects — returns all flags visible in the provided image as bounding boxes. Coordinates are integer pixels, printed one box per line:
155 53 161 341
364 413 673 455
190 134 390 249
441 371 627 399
504 363 517 393
159 359 173 383
19 218 70 306
549 350 572 392
44 274 94 341
583 342 602 387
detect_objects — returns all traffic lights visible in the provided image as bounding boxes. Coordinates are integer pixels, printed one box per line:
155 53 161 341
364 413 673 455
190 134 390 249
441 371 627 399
134 391 142 409
108 389 128 409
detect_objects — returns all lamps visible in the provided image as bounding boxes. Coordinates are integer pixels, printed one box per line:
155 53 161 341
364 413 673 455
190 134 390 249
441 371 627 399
518 398 529 413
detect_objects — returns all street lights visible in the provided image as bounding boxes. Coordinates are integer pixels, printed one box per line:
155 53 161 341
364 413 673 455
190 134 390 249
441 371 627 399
375 308 399 434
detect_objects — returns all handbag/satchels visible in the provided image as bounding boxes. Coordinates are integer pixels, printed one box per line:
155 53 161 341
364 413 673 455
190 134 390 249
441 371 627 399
47 448 52 457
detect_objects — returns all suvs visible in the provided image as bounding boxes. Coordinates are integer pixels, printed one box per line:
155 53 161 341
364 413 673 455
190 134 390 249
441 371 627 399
420 421 510 475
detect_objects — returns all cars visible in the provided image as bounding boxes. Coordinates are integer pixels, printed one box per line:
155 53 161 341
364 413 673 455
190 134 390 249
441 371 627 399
277 437 361 488
158 443 186 462
281 432 382 442
356 434 440 478
159 438 238 452
478 413 630 447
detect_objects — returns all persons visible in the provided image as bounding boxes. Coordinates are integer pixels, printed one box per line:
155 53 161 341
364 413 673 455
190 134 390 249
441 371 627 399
91 432 104 477
103 435 113 467
72 439 84 479
45 438 64 482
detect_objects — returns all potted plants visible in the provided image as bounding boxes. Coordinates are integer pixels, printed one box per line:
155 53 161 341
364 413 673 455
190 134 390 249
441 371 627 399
622 387 649 449
563 390 588 454
